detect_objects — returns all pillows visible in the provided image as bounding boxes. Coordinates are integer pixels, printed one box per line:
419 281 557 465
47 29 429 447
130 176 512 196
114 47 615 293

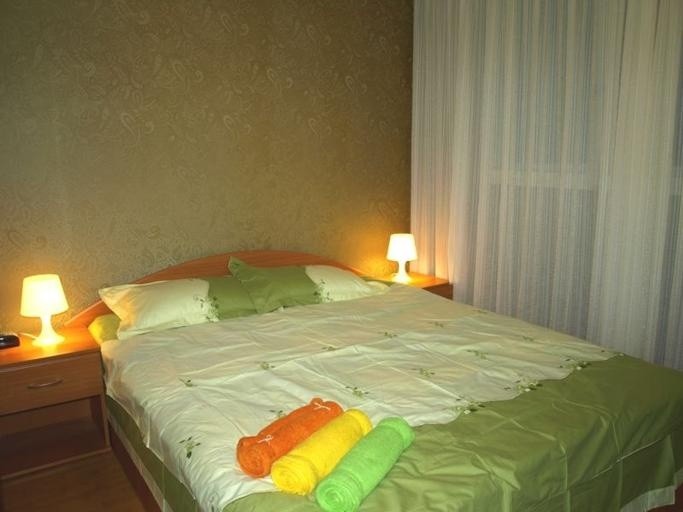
227 256 390 315
98 274 286 341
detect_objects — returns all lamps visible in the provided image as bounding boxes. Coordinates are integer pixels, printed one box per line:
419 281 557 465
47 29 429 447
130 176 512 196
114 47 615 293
19 273 70 348
386 233 418 285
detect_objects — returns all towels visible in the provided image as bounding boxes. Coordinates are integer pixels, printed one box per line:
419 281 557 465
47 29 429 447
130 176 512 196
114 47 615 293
269 408 373 495
314 417 415 511
236 395 344 479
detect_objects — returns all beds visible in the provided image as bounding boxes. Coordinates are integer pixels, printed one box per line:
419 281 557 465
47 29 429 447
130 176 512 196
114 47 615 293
59 250 683 512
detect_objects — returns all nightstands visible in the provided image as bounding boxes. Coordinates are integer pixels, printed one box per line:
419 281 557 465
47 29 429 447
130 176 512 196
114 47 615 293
407 272 452 301
0 326 112 488
